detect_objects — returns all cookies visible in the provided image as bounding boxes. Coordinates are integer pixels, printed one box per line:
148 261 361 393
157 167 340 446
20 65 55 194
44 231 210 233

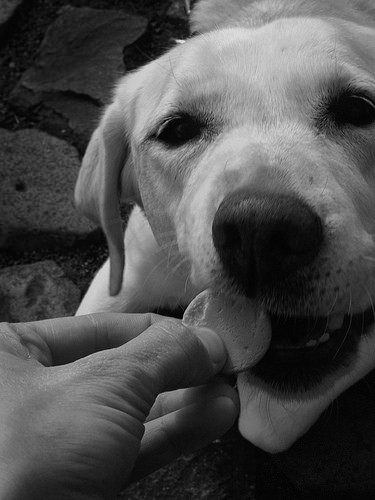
181 289 271 374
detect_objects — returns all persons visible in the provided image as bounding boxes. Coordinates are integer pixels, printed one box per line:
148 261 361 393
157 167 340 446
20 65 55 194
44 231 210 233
0 312 240 500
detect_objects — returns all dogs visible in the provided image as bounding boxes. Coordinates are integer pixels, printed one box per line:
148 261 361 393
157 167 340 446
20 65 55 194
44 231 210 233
74 0 375 455
